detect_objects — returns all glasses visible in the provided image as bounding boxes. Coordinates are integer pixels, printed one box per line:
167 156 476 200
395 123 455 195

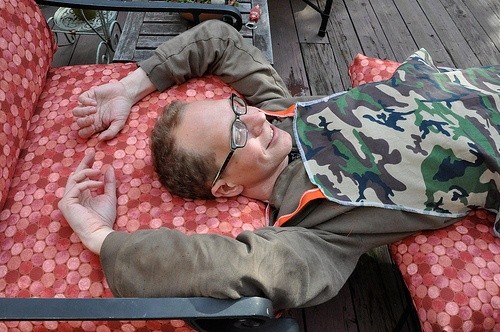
211 92 248 187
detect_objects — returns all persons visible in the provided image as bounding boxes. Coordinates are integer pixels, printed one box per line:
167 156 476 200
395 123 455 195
56 18 499 307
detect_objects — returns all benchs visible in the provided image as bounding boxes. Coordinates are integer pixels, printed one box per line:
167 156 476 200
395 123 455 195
0 1 272 332
104 2 274 67
347 50 500 332
